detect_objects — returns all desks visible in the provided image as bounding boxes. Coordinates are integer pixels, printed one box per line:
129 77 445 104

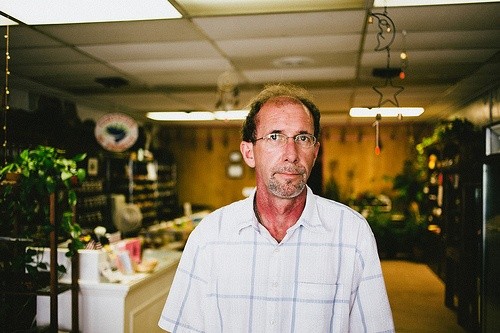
21 246 182 333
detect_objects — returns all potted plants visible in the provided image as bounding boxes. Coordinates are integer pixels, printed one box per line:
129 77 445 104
1 147 87 289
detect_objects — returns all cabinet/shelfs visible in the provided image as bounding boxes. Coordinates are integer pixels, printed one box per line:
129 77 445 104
0 177 79 332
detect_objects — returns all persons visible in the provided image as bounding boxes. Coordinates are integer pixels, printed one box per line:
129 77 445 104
158 85 395 333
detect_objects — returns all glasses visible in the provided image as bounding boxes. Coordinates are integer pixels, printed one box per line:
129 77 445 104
250 133 317 148
